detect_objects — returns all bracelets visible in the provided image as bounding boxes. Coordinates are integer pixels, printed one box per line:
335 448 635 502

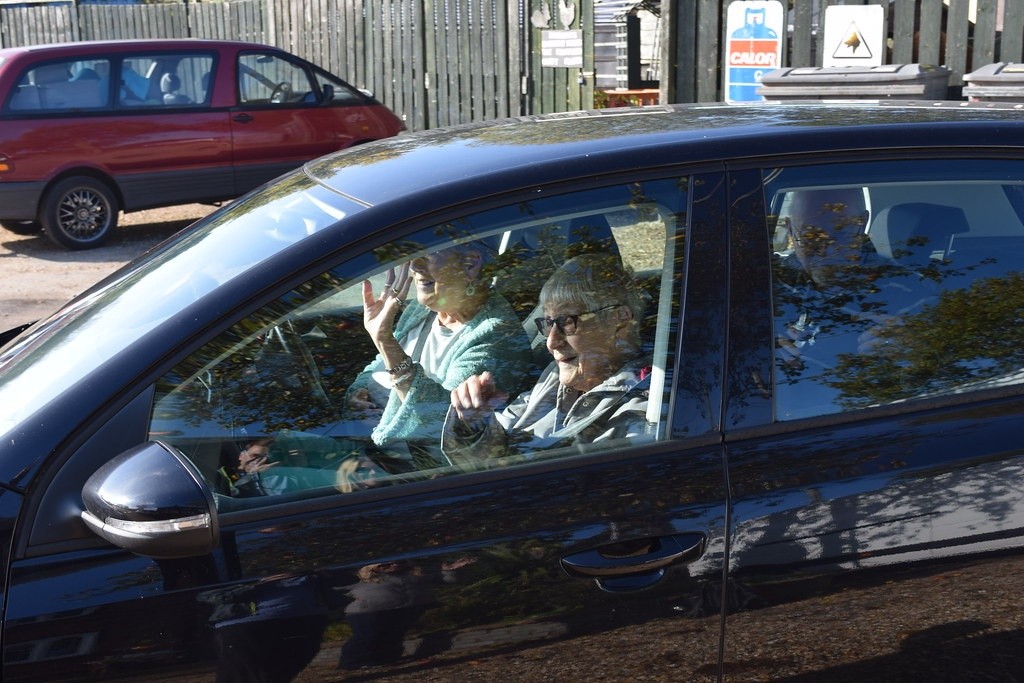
385 355 412 374
392 364 416 388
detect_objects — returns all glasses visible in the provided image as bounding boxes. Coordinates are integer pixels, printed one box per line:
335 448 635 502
534 304 634 337
793 225 865 256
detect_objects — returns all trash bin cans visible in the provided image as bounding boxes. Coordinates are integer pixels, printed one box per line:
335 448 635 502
755 63 952 100
961 62 1024 102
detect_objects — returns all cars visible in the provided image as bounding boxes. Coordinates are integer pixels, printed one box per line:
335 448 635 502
0 36 408 249
0 96 1024 683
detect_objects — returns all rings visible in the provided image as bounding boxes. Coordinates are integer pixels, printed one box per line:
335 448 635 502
885 338 888 347
385 284 391 287
396 297 404 305
393 288 399 294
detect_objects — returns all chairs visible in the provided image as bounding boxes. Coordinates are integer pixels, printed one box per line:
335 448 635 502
865 202 970 276
492 213 623 326
201 72 211 90
160 73 192 105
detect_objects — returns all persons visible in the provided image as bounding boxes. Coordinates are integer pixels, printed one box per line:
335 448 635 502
211 439 376 683
442 254 653 468
258 235 534 495
338 558 475 670
773 188 941 408
